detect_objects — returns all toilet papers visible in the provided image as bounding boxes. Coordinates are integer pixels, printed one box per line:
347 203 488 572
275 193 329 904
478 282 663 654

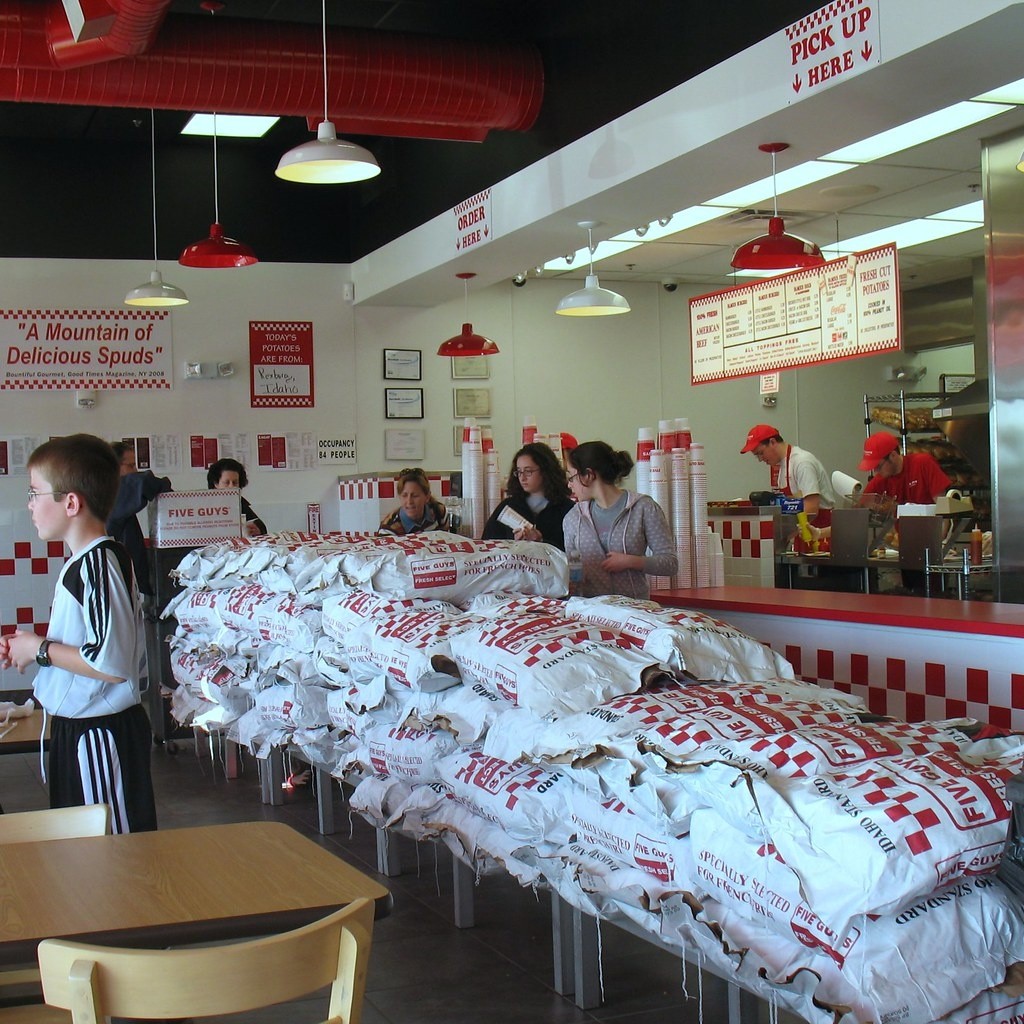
831 470 863 505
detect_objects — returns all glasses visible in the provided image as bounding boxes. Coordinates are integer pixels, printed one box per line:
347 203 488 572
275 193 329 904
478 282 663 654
513 469 538 478
567 472 579 481
874 461 887 471
399 468 429 483
754 445 769 455
27 489 66 500
121 462 137 469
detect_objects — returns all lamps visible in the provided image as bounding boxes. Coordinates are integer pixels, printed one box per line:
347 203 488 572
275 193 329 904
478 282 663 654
184 360 235 380
124 108 190 306
435 273 500 356
275 0 381 185
880 364 928 382
178 112 259 268
555 221 631 317
730 142 825 271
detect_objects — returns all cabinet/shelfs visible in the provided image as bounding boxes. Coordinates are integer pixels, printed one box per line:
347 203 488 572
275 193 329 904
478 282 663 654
863 390 976 495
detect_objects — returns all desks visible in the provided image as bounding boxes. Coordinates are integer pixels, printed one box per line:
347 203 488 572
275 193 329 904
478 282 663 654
0 821 395 1024
195 717 1024 1024
0 709 52 754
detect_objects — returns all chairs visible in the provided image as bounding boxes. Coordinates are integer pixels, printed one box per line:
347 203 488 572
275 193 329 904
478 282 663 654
0 804 112 1024
37 899 376 1024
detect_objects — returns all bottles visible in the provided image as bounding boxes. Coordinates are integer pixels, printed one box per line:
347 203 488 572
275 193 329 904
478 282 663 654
972 523 983 565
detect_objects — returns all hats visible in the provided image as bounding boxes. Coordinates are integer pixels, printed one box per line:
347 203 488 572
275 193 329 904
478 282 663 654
856 432 898 470
738 424 778 453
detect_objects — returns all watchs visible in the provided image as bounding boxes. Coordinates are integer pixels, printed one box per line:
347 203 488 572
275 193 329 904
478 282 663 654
36 638 55 667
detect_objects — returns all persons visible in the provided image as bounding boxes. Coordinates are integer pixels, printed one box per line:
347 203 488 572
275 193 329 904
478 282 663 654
0 435 158 835
481 442 576 552
207 458 267 538
562 441 679 599
740 425 834 550
795 432 954 547
107 441 174 594
378 468 449 536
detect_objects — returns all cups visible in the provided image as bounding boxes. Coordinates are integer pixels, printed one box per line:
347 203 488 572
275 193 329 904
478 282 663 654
635 417 725 590
461 417 502 540
522 414 564 469
